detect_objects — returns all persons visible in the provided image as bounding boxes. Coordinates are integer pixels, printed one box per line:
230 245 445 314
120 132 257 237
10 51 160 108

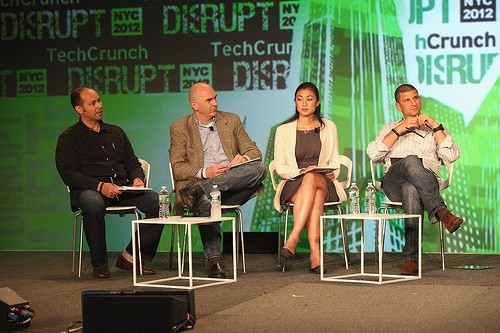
366 84 463 274
273 82 347 274
169 83 268 277
55 86 173 278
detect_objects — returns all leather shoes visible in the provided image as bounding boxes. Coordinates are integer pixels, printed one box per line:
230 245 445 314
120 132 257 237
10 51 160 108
208 256 226 278
115 253 157 275
93 265 110 278
178 187 199 210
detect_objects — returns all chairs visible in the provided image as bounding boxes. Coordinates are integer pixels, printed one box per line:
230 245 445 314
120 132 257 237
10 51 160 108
66 158 150 277
169 161 246 275
269 155 352 272
370 151 453 271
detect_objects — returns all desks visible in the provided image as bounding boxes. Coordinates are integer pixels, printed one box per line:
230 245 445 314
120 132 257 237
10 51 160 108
132 215 237 290
320 214 422 284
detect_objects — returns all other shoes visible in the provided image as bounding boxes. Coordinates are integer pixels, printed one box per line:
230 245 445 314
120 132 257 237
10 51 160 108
400 260 418 275
310 259 327 274
435 208 463 233
280 245 296 258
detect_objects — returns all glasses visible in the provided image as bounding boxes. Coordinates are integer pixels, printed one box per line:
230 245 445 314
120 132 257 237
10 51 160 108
405 122 433 138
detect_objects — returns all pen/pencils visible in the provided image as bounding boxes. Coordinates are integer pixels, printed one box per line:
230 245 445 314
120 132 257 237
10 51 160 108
110 176 119 201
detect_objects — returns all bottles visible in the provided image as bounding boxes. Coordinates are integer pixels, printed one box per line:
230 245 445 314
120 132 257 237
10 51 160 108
210 185 222 219
365 183 376 215
349 182 360 214
158 186 169 219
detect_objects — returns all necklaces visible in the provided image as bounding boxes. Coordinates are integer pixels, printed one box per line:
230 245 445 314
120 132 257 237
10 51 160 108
299 120 314 134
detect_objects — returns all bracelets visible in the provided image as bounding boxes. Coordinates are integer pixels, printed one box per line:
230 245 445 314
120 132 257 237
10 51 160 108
392 129 400 137
100 182 104 194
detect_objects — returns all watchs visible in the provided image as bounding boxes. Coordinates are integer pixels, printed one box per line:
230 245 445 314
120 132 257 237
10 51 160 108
433 124 445 133
